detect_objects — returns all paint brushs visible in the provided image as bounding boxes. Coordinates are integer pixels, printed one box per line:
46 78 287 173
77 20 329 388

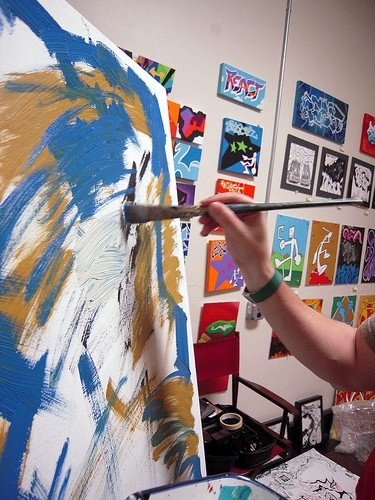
124 196 364 224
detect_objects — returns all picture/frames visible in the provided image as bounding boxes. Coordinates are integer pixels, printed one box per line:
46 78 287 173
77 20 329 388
279 134 319 196
316 147 349 200
294 394 323 456
327 293 375 439
267 298 324 360
346 157 374 208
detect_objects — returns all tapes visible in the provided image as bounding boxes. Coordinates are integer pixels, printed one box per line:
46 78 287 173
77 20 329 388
219 413 243 431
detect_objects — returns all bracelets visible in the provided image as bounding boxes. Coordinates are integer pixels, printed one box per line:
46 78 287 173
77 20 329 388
242 268 283 303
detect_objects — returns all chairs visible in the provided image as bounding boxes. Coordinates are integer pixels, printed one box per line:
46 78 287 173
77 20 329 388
191 331 299 482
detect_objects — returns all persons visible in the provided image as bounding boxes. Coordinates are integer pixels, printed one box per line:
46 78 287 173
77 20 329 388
198 192 375 500
